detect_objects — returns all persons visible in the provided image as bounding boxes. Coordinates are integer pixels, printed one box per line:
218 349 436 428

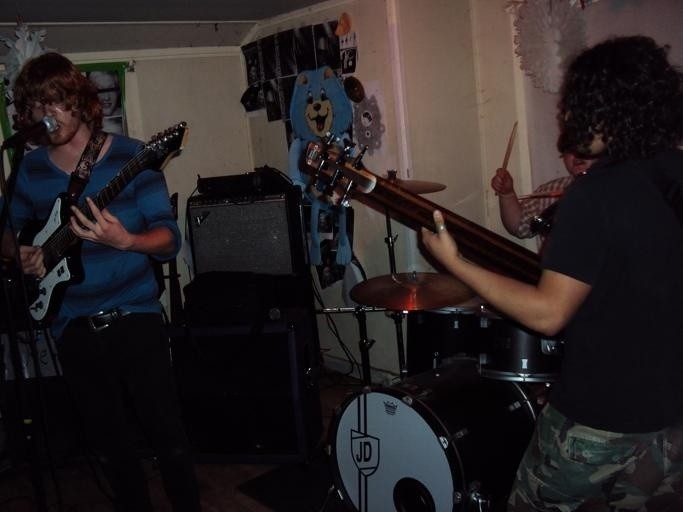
87 70 123 120
491 123 610 255
422 37 683 512
2 53 204 511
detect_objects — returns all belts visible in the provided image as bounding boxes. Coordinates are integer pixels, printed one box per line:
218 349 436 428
83 308 132 332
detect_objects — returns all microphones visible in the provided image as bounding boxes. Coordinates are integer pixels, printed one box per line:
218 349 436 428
2 115 58 151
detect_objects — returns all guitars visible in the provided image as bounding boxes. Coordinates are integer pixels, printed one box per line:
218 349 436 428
297 130 544 287
5 120 188 328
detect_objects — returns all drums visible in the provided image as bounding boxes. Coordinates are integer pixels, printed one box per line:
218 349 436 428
477 316 572 383
324 355 538 512
406 307 482 380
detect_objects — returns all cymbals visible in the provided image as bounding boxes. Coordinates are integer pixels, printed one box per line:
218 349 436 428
516 190 567 201
349 271 474 312
386 168 447 195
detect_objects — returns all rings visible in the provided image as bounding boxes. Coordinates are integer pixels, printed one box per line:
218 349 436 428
434 224 447 231
